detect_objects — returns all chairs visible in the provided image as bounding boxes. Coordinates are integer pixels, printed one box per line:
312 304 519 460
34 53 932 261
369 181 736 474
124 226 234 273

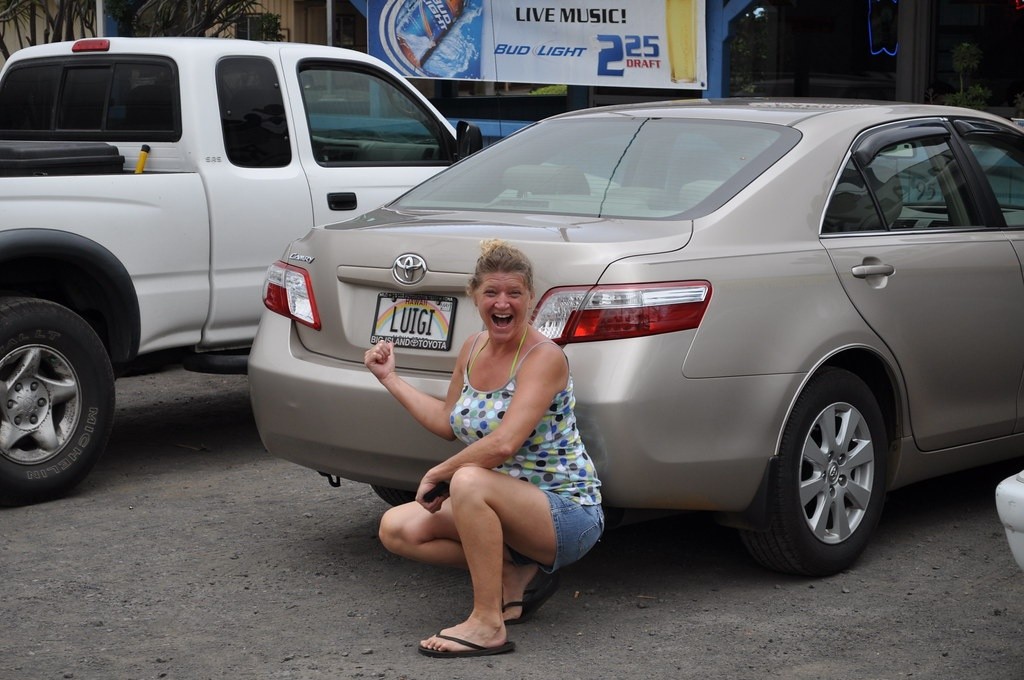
137 73 175 118
70 84 163 131
657 167 730 211
31 73 94 134
623 149 678 194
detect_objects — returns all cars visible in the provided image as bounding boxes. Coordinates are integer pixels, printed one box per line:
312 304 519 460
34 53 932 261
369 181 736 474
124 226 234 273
246 96 1024 582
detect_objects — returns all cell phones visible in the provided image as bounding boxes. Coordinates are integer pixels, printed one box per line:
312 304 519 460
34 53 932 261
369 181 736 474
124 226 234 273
422 483 449 503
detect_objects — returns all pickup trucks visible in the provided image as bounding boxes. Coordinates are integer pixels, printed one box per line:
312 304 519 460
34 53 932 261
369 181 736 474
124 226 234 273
0 37 621 508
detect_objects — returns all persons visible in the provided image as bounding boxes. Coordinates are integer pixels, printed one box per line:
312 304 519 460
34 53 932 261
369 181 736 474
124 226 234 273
364 239 604 659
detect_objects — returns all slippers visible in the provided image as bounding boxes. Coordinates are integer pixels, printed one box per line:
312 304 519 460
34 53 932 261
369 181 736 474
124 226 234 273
502 574 558 625
418 629 515 658
